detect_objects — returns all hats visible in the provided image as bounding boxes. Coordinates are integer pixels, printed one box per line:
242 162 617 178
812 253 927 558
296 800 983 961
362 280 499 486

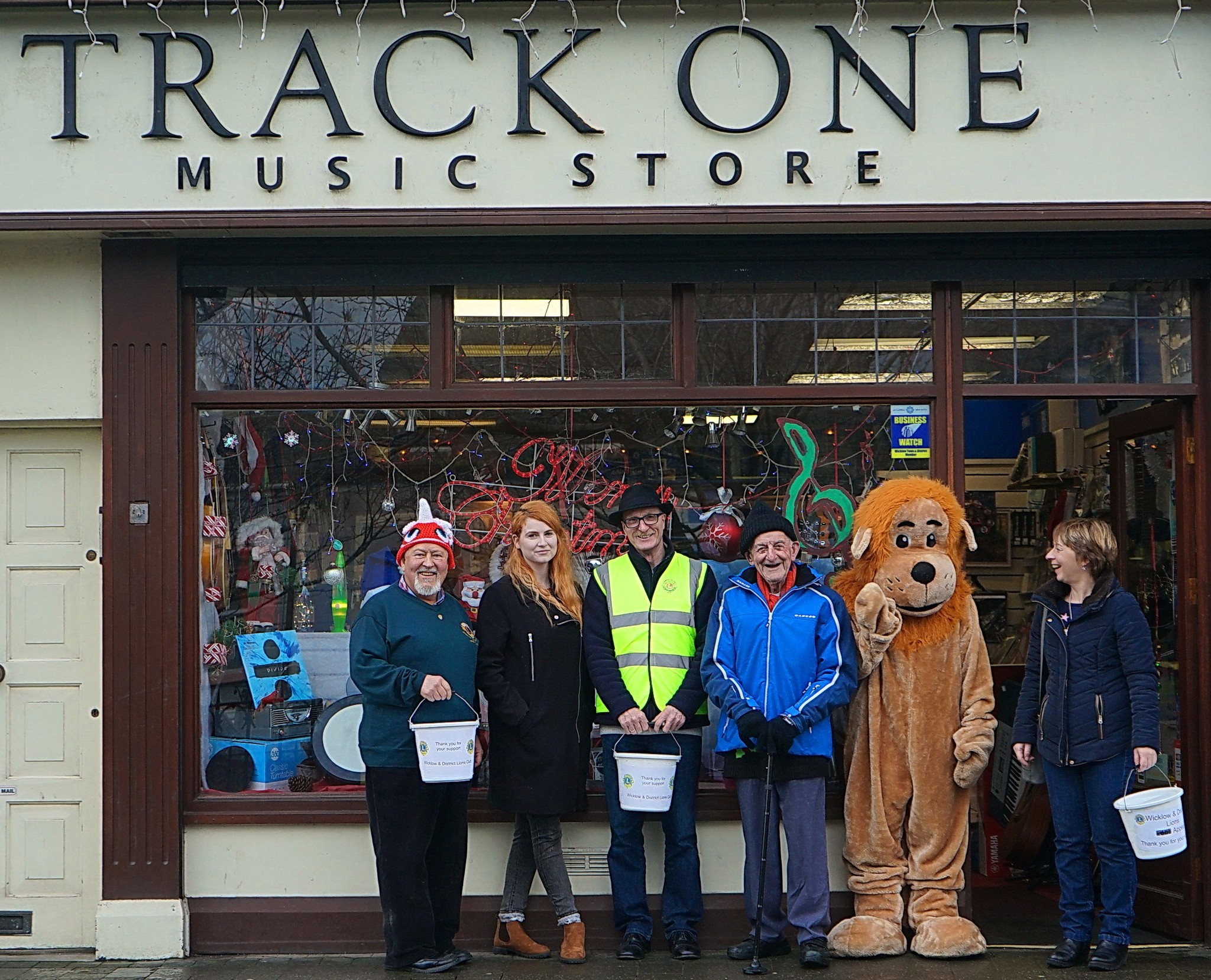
396 498 458 572
608 483 675 529
739 497 798 556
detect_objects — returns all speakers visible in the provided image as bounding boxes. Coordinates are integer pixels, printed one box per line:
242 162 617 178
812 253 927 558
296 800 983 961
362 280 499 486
1028 432 1056 476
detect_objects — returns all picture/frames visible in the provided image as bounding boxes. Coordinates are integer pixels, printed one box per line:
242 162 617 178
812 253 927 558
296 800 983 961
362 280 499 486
966 511 1011 567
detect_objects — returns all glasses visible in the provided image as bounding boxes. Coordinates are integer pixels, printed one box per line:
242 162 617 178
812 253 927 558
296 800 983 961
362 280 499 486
621 512 664 531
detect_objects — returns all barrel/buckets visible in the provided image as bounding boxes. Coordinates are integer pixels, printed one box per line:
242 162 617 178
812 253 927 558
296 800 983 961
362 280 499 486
613 722 682 813
1113 763 1187 859
409 690 480 783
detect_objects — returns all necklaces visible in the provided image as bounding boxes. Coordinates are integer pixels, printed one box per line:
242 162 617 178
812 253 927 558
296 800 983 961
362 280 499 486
1068 592 1073 623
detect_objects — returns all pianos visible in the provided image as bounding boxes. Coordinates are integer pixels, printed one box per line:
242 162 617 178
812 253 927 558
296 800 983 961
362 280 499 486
987 698 1034 828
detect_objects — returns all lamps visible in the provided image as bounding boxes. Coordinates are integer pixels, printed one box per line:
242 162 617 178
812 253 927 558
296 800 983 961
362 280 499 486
342 382 418 433
664 407 748 448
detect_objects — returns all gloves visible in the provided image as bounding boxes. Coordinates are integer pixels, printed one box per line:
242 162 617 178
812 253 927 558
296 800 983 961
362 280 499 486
737 708 779 758
755 715 800 763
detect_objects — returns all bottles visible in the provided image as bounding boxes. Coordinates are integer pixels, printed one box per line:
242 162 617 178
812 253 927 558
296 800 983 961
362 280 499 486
1009 441 1029 482
323 561 344 586
830 515 848 572
1013 515 1036 546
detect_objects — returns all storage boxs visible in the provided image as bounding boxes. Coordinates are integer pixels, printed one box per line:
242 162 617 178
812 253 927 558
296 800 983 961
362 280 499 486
258 565 274 579
204 587 222 603
203 461 219 479
202 515 226 537
203 643 323 791
1008 399 1085 482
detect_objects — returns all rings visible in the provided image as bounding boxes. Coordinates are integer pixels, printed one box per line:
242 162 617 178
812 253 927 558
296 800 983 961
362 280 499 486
628 729 635 732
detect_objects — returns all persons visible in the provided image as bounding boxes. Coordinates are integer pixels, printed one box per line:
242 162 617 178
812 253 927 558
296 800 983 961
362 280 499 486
585 484 718 961
238 517 290 632
458 574 485 631
475 500 587 964
349 499 483 973
1011 517 1160 972
699 498 859 968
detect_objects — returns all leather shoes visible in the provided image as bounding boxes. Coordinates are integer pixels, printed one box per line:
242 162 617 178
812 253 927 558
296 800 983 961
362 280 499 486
398 948 463 972
667 928 703 959
436 947 474 962
1044 938 1093 969
726 935 791 961
615 931 652 959
797 936 833 968
1087 938 1129 971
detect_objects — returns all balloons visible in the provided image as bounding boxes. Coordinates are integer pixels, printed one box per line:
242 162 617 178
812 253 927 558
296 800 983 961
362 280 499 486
700 505 743 563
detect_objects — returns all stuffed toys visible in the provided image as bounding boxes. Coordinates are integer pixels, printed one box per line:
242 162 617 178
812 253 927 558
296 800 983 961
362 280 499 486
826 476 998 957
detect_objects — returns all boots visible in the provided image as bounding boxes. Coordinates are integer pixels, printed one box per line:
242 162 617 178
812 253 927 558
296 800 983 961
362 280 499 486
492 913 553 959
559 920 587 964
